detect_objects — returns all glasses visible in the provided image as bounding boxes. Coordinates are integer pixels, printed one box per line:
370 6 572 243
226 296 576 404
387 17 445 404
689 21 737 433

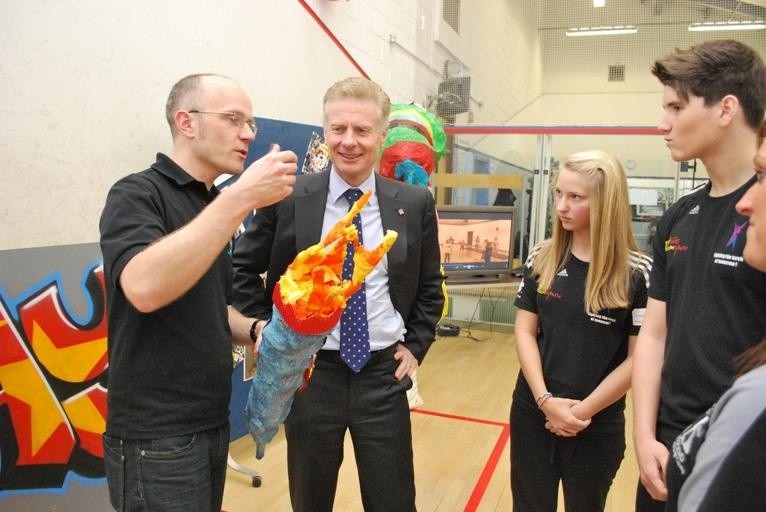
186 109 261 143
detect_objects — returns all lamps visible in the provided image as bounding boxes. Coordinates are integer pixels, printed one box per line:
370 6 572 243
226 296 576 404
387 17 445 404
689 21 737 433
688 21 766 31
566 25 638 37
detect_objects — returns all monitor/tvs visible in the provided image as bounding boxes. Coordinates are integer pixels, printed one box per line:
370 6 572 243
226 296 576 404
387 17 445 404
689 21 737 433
436 205 517 285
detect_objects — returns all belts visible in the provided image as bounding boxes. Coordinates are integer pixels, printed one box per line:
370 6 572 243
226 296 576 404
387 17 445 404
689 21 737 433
317 343 402 364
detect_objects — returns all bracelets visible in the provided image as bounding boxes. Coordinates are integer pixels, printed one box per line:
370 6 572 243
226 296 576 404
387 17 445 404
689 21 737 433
536 392 554 410
250 318 262 344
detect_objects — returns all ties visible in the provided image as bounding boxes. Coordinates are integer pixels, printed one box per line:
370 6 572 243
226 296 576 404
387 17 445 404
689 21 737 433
338 189 371 375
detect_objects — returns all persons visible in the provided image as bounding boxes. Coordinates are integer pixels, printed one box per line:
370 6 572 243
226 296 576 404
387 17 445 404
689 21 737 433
440 235 502 263
95 73 299 512
661 118 766 512
303 143 325 171
508 149 652 510
235 75 445 511
627 42 766 512
